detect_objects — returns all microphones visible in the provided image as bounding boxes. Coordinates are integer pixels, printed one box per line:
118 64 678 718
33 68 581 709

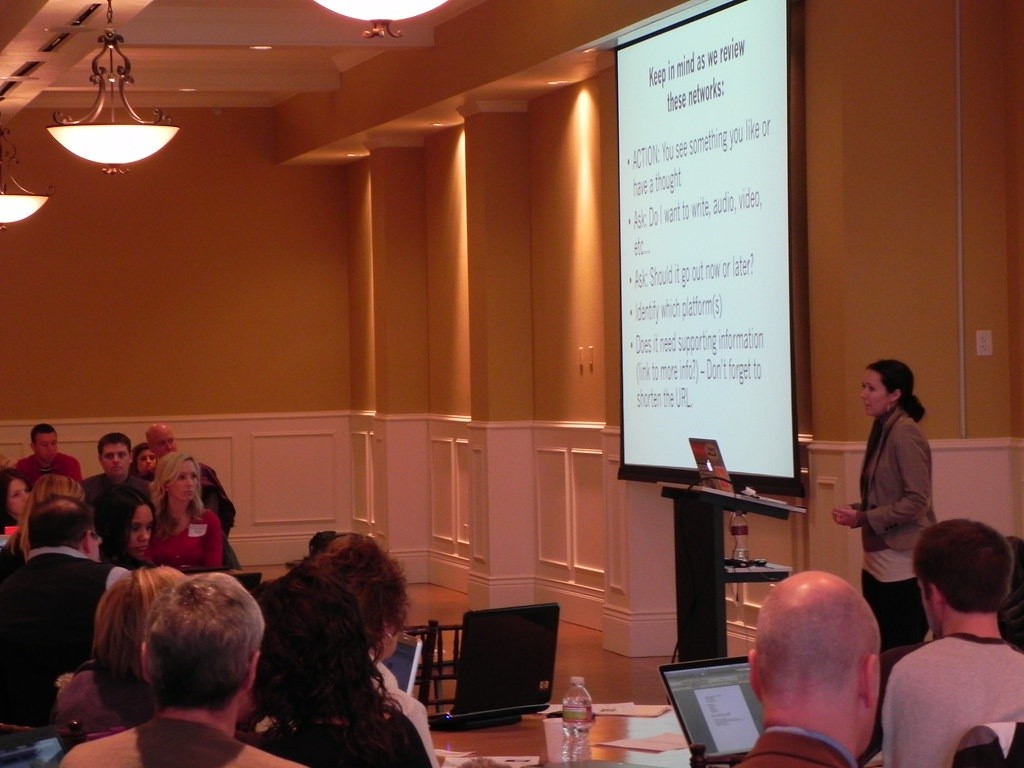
689 476 746 491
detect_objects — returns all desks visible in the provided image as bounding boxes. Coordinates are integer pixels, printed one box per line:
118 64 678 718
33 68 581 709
412 699 735 768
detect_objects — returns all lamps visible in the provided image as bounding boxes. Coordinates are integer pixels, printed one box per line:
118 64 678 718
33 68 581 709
308 0 446 43
0 109 56 231
48 1 183 176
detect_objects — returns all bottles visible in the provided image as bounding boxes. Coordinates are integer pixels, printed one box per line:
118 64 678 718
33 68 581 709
561 676 593 762
730 511 750 566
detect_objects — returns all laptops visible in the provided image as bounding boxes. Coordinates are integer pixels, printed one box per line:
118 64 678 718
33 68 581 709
427 602 560 725
688 437 787 504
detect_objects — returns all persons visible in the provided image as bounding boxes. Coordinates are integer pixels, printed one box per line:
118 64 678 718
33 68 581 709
55 562 188 754
0 468 33 535
0 496 132 728
129 441 158 481
881 517 1024 768
238 532 442 768
84 432 148 505
265 574 434 768
831 359 938 653
145 422 235 538
145 451 223 572
15 423 83 487
0 474 85 585
997 535 1023 652
93 484 157 570
731 570 881 768
55 572 310 768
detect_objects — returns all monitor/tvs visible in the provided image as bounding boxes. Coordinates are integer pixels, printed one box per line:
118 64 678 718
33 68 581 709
383 631 422 696
657 656 766 766
